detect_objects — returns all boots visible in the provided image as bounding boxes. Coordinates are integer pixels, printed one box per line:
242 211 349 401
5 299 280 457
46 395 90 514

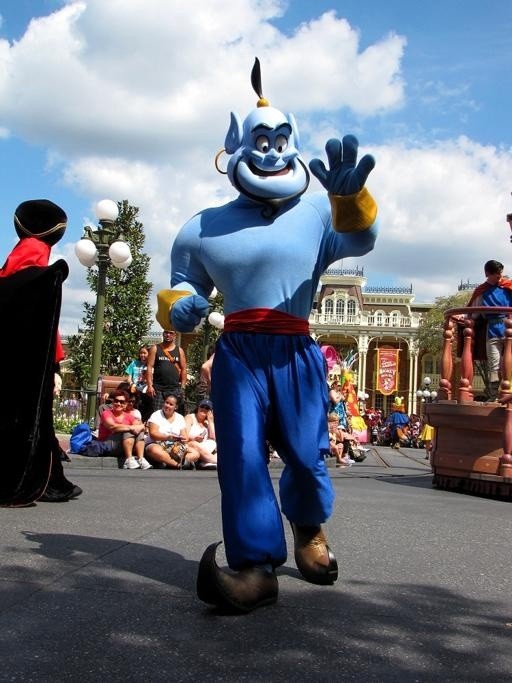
487 381 499 402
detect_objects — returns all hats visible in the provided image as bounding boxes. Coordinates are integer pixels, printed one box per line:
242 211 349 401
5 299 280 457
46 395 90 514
199 399 212 410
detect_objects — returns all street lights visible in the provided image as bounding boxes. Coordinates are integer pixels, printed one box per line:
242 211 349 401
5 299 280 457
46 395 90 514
201 286 225 363
416 376 438 403
357 390 370 413
76 196 133 432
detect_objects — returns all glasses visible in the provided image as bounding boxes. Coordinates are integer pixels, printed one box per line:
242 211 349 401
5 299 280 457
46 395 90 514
114 398 125 404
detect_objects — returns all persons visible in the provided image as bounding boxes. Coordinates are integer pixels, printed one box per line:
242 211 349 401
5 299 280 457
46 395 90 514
95 329 219 472
0 198 83 510
328 378 370 465
155 55 380 615
457 259 512 402
418 424 434 459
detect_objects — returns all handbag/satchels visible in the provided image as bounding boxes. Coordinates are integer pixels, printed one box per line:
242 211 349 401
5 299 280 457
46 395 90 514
70 424 113 456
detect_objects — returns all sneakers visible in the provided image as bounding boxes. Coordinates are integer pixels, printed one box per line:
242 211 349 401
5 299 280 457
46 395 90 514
124 457 141 469
138 457 153 470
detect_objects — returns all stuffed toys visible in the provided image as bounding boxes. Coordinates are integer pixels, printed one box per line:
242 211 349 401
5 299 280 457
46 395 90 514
385 396 409 449
